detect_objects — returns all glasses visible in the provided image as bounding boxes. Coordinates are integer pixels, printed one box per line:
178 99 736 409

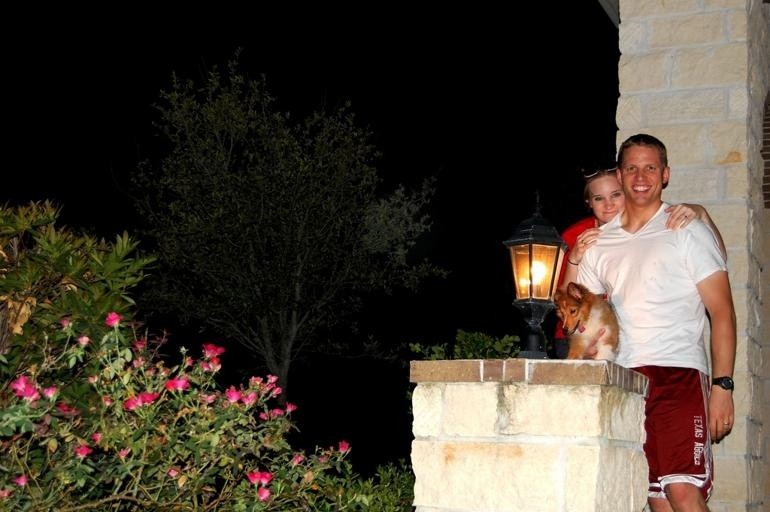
583 161 619 178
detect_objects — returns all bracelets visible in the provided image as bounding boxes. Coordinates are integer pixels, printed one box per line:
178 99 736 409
567 257 578 266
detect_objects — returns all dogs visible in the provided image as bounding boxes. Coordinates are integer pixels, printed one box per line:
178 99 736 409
554 281 620 362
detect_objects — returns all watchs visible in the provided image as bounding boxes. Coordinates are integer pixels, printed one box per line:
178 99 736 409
711 375 734 391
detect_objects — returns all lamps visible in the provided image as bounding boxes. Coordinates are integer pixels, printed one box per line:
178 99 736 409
503 193 570 360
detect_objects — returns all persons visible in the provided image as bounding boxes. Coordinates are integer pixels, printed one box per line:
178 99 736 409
550 160 728 360
574 133 738 510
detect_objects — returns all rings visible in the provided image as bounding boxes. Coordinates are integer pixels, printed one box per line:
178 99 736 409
683 214 688 218
